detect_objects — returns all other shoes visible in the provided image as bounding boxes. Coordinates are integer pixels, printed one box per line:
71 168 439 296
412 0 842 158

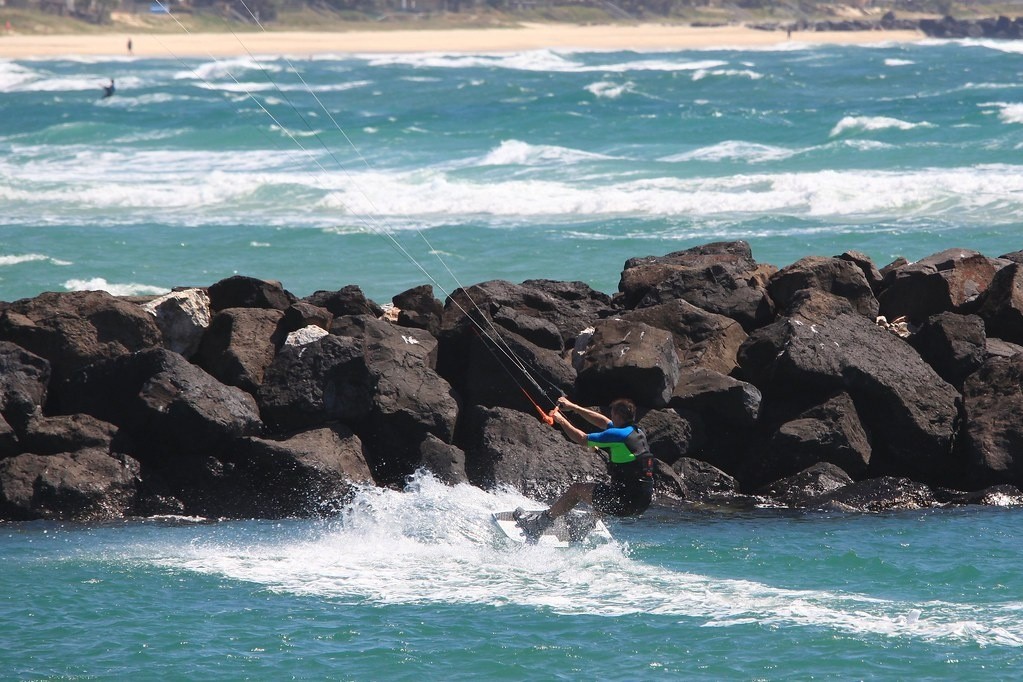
566 510 598 543
513 507 554 545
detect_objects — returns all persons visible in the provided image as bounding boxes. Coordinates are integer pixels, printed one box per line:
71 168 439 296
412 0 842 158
102 78 115 99
128 39 132 52
6 20 10 34
788 28 790 37
515 396 653 540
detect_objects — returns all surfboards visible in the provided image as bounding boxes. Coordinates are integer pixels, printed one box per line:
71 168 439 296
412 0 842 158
495 506 599 549
